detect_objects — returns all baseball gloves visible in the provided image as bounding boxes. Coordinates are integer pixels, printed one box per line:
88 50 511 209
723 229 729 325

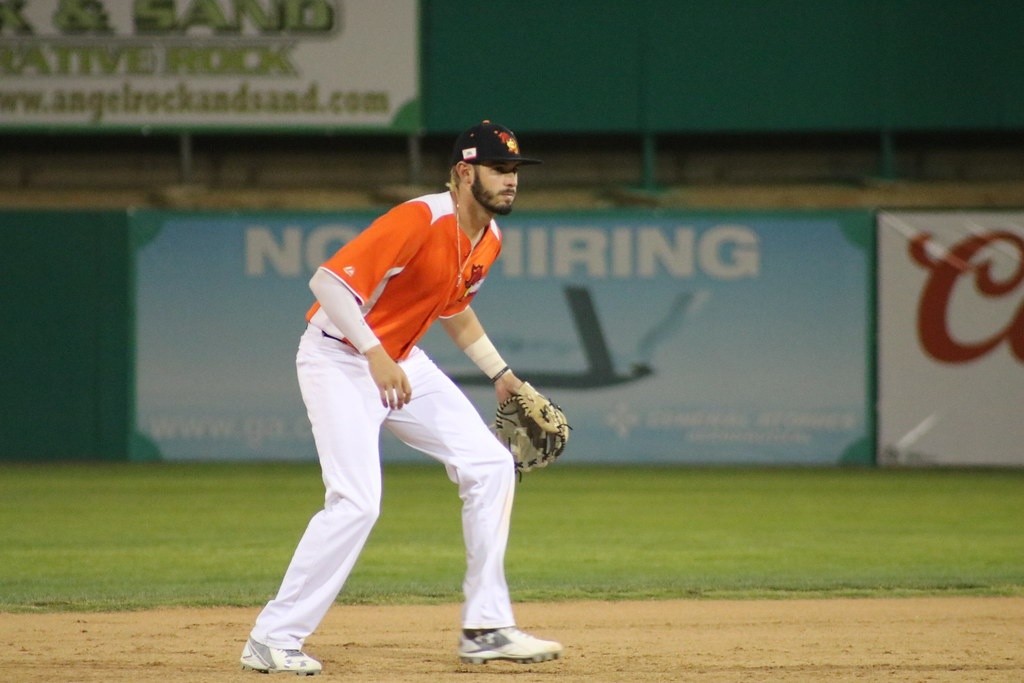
492 379 571 471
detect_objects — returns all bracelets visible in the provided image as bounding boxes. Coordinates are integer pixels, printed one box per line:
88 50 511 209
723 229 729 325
463 333 509 385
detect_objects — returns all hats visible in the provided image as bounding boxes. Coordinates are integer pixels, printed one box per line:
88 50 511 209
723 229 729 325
455 119 545 165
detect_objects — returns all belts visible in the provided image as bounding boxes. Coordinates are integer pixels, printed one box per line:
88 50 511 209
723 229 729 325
306 324 344 342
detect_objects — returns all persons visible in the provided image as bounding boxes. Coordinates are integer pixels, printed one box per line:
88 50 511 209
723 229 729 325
240 121 572 676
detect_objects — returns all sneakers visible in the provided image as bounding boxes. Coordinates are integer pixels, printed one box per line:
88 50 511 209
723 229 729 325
239 634 322 676
457 627 563 664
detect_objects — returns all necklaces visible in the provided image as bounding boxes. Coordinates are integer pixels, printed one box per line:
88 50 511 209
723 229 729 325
456 202 481 287
